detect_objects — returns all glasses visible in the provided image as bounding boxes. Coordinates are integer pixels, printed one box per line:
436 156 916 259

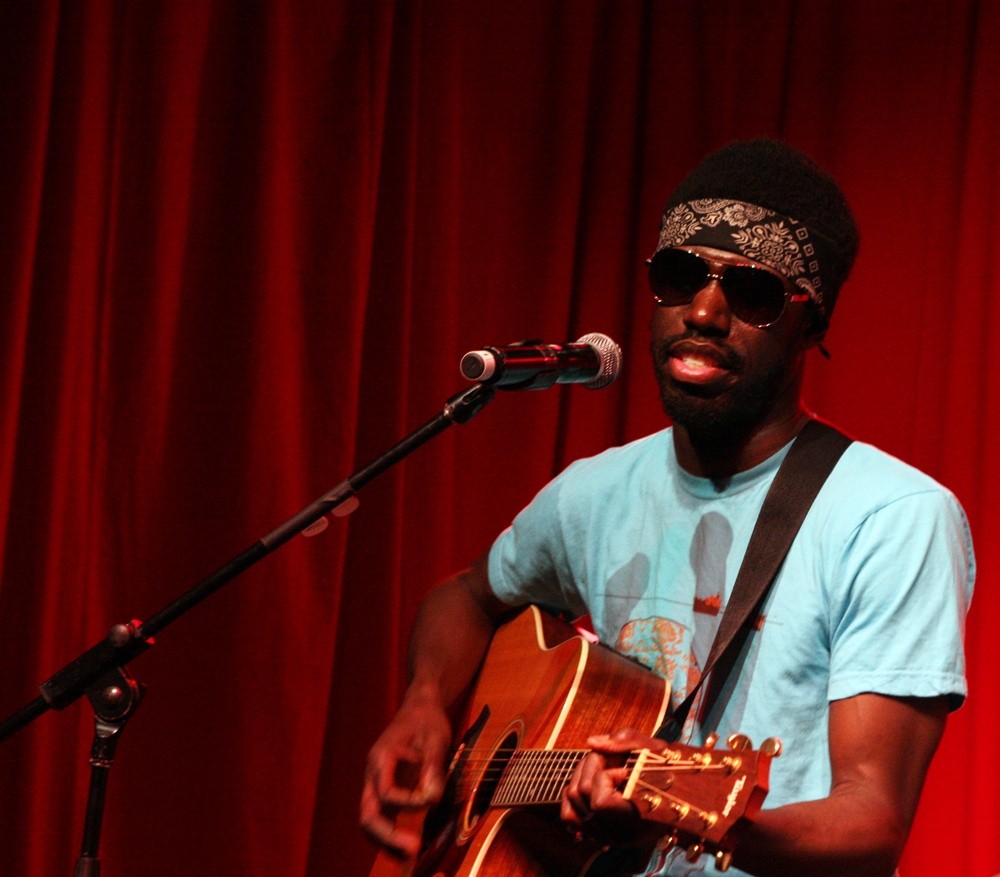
645 246 810 330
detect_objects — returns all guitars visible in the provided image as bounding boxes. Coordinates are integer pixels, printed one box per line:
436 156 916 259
370 601 784 875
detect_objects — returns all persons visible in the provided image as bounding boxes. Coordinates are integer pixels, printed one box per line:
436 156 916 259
358 142 977 877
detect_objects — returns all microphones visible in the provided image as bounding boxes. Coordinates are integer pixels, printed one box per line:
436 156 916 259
457 331 624 393
